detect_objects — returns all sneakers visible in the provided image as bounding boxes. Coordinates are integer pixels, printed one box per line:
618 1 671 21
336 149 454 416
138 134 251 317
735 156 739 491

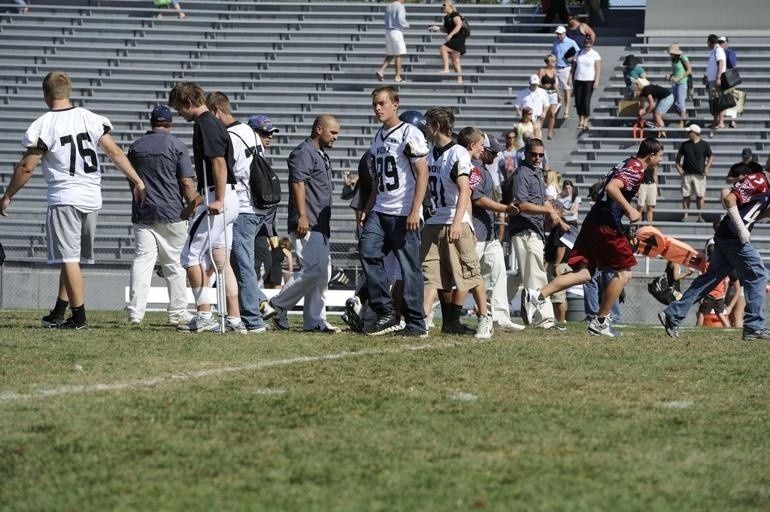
658 311 679 338
341 287 624 339
743 326 770 341
41 310 91 332
176 301 342 332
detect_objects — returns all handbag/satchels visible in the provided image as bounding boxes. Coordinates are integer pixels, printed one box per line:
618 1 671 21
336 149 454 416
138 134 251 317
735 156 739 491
688 73 692 88
648 275 676 304
722 67 742 90
460 19 470 37
709 93 736 114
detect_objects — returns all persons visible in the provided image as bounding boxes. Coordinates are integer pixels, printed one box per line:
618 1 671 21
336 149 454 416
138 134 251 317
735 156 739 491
123 80 341 334
429 0 469 83
343 86 767 342
375 2 410 82
1 70 148 331
514 18 738 141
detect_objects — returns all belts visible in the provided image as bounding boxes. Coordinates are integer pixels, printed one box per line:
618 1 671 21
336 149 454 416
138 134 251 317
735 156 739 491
203 185 235 195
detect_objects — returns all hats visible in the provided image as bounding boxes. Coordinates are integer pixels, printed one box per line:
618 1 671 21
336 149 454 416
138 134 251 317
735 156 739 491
554 26 566 34
742 148 752 157
666 45 683 55
718 36 726 41
483 133 505 153
622 55 640 66
529 74 540 85
248 114 280 133
683 124 702 134
151 106 172 119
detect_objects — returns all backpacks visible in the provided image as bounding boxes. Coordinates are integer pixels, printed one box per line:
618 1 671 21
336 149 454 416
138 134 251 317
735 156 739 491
228 127 281 209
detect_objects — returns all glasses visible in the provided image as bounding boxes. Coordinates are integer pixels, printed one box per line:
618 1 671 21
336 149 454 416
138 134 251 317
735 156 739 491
526 150 544 158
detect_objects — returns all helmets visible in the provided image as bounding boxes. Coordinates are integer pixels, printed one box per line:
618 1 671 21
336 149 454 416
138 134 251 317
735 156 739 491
399 110 427 134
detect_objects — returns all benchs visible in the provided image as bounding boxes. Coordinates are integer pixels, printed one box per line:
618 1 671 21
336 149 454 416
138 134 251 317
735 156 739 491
0 0 769 286
